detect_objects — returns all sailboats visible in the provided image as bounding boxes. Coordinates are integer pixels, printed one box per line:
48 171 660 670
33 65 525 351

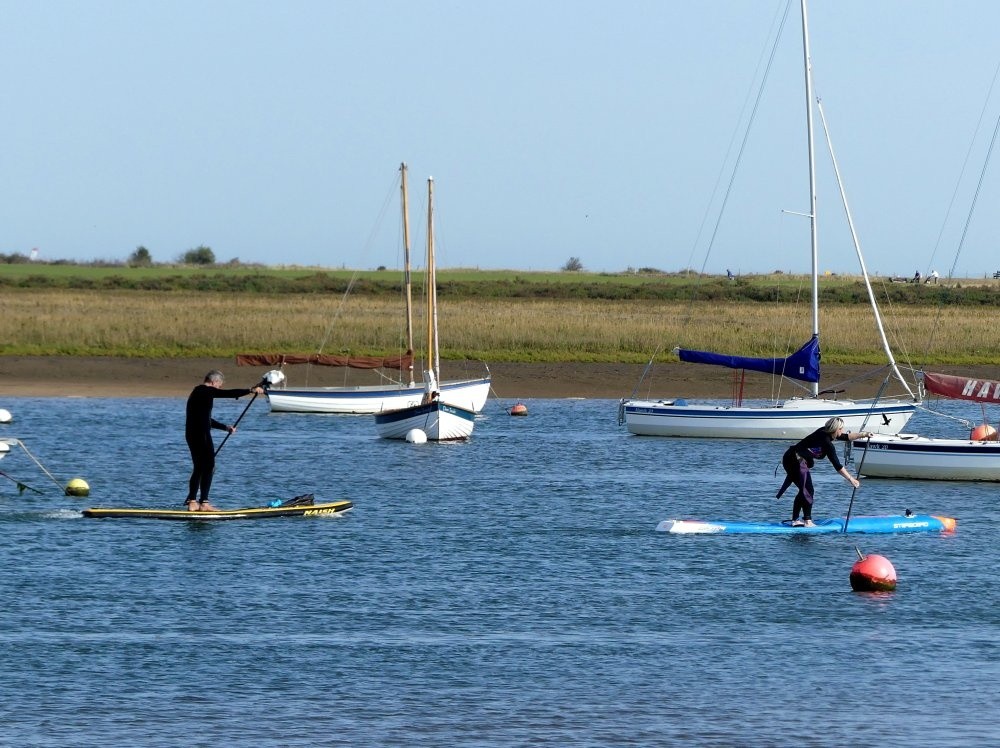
371 175 477 441
617 0 928 440
261 160 493 416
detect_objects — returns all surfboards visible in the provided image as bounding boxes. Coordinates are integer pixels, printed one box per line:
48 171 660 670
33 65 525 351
655 515 958 537
83 498 354 522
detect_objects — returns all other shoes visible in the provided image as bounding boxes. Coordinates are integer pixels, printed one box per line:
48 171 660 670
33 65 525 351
792 520 804 526
804 520 816 527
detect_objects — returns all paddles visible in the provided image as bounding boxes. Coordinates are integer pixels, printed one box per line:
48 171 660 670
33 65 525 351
214 369 286 454
844 436 871 533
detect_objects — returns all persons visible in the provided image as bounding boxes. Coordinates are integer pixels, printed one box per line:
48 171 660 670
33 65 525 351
727 270 733 280
931 269 938 284
911 270 919 283
776 417 873 527
186 369 264 511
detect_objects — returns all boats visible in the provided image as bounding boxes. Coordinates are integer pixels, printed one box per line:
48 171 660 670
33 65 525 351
851 427 999 482
